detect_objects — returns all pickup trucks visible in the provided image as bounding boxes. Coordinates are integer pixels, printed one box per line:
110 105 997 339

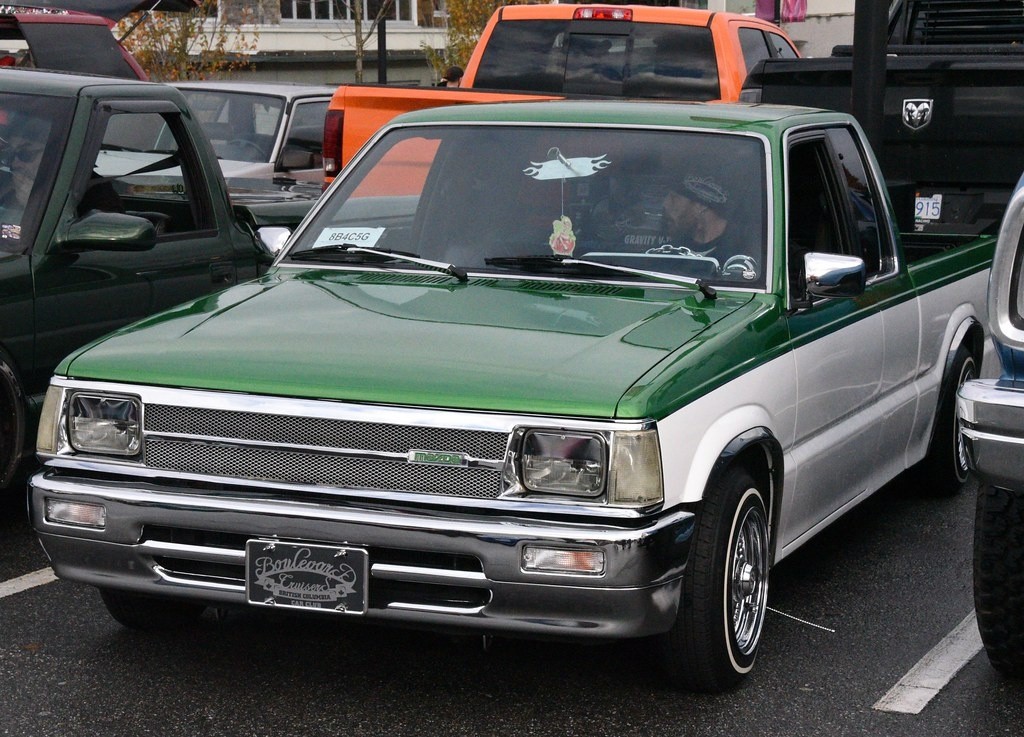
313 3 801 211
738 1 1024 238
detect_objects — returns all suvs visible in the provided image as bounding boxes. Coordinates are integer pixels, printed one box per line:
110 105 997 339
0 0 203 137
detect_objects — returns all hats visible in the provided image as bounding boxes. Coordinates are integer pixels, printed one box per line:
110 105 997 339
668 165 740 218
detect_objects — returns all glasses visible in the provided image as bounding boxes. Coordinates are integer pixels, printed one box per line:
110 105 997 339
0 142 45 166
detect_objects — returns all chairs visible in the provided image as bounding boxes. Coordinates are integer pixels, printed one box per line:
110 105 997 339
127 210 172 236
278 150 316 169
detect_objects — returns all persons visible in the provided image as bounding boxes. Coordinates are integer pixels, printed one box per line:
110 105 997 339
444 66 463 88
0 116 123 218
660 167 760 262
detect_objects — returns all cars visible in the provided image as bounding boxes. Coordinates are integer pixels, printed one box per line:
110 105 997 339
24 95 1009 696
81 81 350 209
944 171 1024 678
0 69 283 501
